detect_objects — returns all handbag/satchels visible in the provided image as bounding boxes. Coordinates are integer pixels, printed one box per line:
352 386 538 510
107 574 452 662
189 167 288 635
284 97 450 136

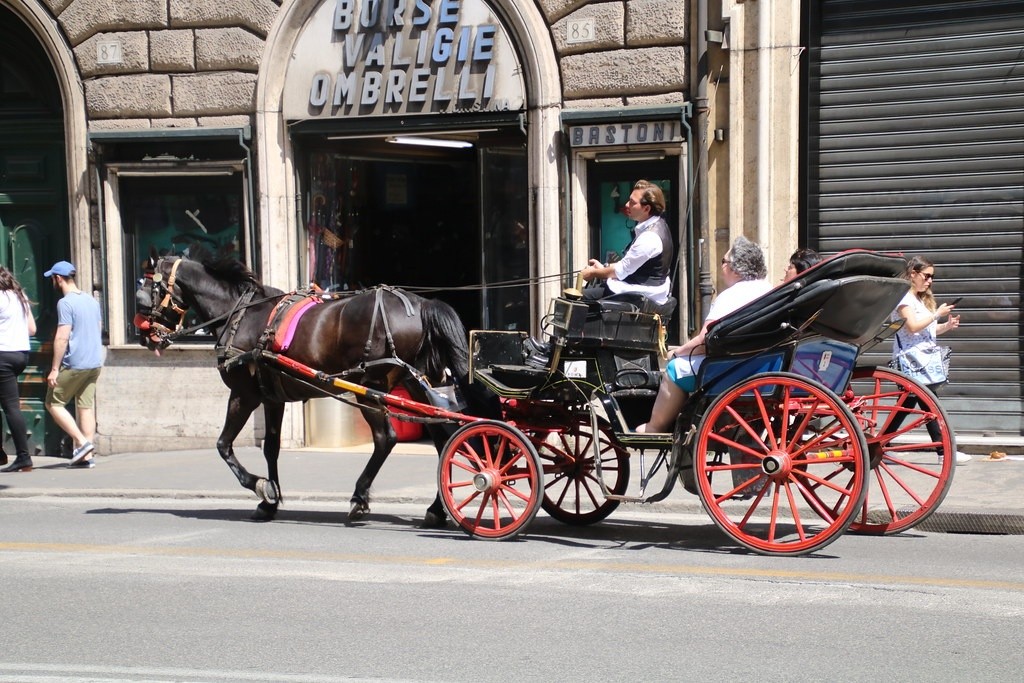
888 345 951 397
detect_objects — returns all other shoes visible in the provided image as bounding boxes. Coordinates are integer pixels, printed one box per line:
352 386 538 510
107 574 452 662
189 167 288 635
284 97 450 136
619 423 641 432
67 459 95 468
883 452 904 464
70 441 92 466
939 451 972 465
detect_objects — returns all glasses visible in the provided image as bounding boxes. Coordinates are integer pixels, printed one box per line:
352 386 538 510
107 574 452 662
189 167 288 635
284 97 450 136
919 271 935 279
722 259 729 264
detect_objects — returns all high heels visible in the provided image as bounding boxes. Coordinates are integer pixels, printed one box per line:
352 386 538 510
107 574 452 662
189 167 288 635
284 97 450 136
0 455 7 465
1 459 33 471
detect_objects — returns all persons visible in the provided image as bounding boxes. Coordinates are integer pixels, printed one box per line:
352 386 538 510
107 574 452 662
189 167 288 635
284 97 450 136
876 256 972 464
783 248 823 284
629 235 774 433
0 264 37 472
43 261 104 469
577 181 674 303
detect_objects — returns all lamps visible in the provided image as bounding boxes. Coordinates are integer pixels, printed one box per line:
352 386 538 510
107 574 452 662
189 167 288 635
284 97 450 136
541 288 589 373
385 136 474 149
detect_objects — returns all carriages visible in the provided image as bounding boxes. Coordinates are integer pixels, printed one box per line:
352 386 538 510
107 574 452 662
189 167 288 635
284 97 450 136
128 229 958 558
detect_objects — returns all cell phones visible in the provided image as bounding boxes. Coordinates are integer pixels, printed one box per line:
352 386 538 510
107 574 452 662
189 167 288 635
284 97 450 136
951 297 962 305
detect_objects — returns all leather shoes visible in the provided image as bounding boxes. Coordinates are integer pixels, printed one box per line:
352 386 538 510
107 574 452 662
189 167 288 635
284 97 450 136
526 356 552 369
524 334 552 354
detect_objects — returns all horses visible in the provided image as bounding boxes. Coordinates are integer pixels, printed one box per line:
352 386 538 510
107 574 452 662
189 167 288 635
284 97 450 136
135 244 473 527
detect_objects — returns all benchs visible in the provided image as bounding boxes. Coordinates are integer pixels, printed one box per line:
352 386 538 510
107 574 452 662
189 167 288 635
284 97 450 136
537 288 680 388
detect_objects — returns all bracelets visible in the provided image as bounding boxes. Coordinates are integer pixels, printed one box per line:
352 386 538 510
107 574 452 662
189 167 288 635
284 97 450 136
604 262 610 268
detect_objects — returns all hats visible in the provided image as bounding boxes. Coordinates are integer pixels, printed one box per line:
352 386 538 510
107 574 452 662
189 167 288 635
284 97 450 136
44 261 74 277
